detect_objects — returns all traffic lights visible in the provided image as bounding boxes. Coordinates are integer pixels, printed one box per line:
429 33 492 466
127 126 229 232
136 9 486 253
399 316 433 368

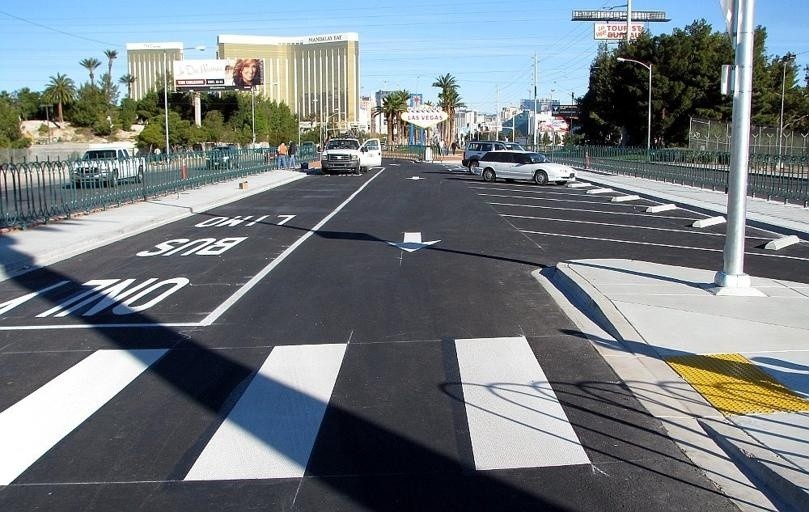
477 123 481 130
467 123 470 130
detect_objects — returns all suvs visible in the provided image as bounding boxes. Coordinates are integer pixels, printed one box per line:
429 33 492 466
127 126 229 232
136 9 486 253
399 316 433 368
321 138 382 175
462 140 525 175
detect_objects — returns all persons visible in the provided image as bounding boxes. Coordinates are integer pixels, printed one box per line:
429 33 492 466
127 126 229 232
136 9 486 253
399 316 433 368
276 140 288 170
436 139 458 156
232 59 260 86
287 142 297 170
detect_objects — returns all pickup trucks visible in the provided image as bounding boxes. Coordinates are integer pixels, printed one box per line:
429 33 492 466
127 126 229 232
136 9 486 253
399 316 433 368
69 147 146 188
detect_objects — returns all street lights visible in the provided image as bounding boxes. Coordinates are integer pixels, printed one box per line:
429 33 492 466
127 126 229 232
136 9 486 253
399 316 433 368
298 99 318 147
38 103 54 143
325 111 345 142
616 58 653 162
163 46 206 166
251 82 280 154
551 86 574 145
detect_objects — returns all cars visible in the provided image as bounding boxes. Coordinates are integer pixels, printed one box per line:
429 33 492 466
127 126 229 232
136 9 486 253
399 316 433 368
204 146 242 170
287 144 291 149
263 149 280 164
299 140 316 157
318 145 325 152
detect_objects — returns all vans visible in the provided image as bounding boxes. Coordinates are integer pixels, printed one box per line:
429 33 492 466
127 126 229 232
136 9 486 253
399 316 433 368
474 150 577 186
